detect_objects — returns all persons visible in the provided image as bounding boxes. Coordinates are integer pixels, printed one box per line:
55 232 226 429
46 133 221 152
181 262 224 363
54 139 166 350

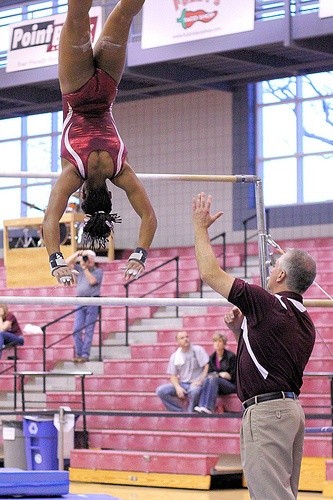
156 330 210 412
66 250 103 362
192 191 317 500
194 332 237 414
42 0 158 285
0 303 23 356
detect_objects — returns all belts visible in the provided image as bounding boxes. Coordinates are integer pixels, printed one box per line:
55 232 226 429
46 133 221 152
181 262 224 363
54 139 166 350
243 392 298 408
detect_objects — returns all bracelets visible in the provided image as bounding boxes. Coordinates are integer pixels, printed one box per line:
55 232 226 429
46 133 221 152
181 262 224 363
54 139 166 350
81 265 86 270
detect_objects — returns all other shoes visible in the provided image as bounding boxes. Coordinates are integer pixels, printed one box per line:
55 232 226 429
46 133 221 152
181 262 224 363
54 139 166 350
73 358 78 362
194 407 201 413
201 407 212 414
78 358 88 361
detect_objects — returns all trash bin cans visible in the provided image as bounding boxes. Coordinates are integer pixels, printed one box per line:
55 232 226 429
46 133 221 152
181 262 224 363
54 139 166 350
22 413 70 471
1 420 27 471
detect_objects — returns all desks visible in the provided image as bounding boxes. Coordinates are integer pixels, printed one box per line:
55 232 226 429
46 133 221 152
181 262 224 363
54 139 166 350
13 370 92 449
3 213 114 288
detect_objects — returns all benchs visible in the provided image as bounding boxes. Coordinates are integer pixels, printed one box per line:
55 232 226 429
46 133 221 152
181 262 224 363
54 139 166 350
0 237 333 500
